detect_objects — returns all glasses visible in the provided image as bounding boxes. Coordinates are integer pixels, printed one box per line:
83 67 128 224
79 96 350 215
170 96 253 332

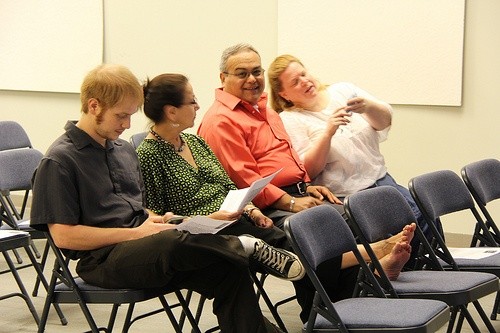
223 68 265 79
177 94 196 105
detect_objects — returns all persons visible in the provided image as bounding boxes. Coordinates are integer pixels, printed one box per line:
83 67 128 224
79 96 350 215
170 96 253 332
197 42 343 324
136 72 416 281
30 62 306 333
267 54 445 258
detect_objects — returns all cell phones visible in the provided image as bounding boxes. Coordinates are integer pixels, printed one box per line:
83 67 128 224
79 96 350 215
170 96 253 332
163 215 188 225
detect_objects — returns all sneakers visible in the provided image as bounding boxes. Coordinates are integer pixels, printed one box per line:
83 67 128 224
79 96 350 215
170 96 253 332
239 233 306 281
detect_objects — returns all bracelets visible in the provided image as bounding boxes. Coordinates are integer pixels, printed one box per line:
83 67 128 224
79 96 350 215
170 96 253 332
289 197 295 212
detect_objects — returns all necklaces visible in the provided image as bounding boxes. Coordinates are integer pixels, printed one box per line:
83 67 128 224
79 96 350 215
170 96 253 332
149 125 186 153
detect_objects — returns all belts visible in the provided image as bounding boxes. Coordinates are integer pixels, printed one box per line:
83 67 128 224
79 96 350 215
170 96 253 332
280 180 308 195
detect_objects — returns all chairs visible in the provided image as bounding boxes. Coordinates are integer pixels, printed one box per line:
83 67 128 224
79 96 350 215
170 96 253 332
0 121 500 333
